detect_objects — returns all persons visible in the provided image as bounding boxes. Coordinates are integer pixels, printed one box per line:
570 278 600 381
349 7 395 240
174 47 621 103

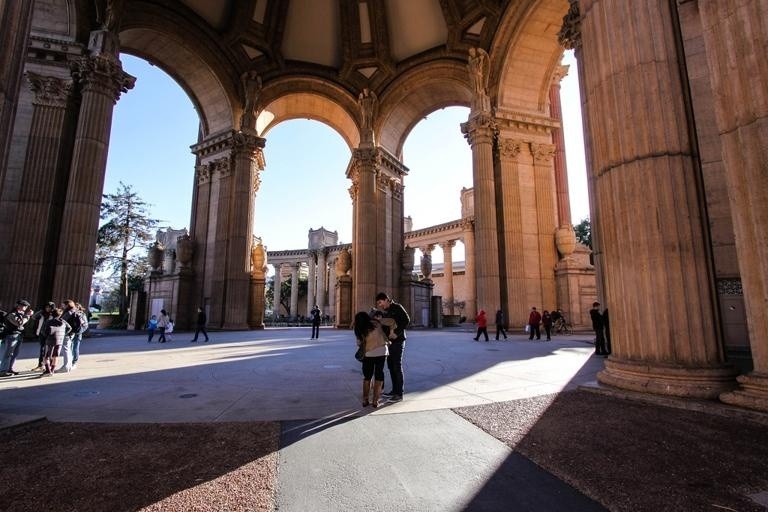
551 308 564 327
353 312 398 407
495 309 508 340
190 306 209 342
310 304 322 340
542 310 552 341
603 305 611 354
589 302 608 355
375 292 410 402
527 306 541 341
0 298 92 377
147 309 174 343
473 308 489 342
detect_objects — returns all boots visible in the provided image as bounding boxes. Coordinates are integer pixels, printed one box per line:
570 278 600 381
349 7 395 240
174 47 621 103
362 379 371 407
373 380 384 408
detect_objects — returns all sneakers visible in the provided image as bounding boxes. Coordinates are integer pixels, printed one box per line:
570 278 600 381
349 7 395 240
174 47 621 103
385 394 403 402
383 391 394 398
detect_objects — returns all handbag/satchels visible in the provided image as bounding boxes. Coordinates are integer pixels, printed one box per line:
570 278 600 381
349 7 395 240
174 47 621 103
355 339 366 361
525 324 530 332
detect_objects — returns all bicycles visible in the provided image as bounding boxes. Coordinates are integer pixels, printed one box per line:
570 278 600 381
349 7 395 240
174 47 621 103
550 317 573 337
